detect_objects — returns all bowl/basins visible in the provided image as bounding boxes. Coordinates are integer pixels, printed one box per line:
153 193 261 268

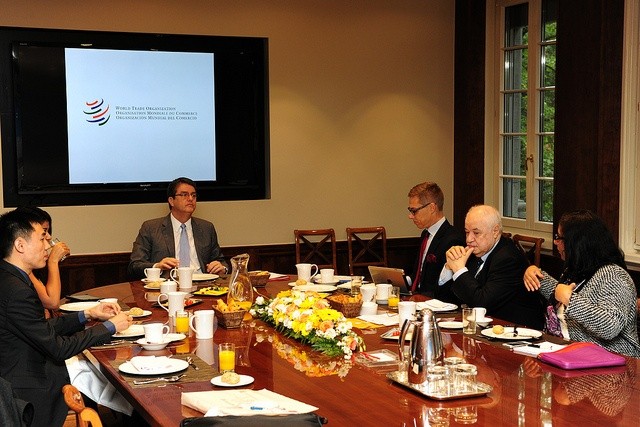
138 342 170 350
466 316 494 328
359 302 378 314
137 350 172 358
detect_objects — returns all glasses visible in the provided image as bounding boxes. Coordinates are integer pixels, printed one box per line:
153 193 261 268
553 232 566 242
170 191 198 197
407 202 435 214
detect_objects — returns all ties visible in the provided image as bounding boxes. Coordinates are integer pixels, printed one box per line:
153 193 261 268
178 223 191 267
471 259 484 279
410 231 430 294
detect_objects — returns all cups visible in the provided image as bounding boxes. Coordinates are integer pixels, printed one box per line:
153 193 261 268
175 333 190 354
144 323 170 340
453 364 478 394
144 292 160 301
170 267 195 289
463 336 476 359
175 310 190 333
462 308 476 334
351 276 362 296
157 292 188 317
376 284 392 304
191 340 215 366
159 279 177 293
428 407 449 427
361 285 376 302
295 263 318 283
188 310 215 339
320 269 335 283
427 366 450 397
453 406 478 424
388 286 400 309
218 343 235 373
49 238 70 261
443 356 466 384
398 301 416 328
118 359 189 376
164 316 178 333
144 268 160 280
473 307 486 317
99 298 118 304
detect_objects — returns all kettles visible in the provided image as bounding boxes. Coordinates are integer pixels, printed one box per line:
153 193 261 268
403 397 442 427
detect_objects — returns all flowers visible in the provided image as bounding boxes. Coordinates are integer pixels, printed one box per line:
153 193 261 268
253 289 364 356
250 319 353 377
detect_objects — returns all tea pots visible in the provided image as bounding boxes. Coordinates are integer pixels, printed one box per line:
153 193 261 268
398 308 443 391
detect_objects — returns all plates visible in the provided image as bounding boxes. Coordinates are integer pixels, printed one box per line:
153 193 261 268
416 301 458 311
314 275 365 284
112 325 145 337
438 321 463 329
191 274 219 280
143 285 160 290
288 282 314 286
136 334 186 342
480 327 542 340
152 299 202 307
210 375 255 386
292 284 337 292
123 309 152 317
141 278 167 284
380 328 411 341
190 286 229 296
59 301 100 311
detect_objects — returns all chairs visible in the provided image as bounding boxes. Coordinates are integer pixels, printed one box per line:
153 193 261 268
347 226 387 275
61 384 102 426
513 235 544 265
294 228 338 275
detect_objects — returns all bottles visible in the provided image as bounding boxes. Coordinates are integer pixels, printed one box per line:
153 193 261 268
227 253 254 310
227 325 254 368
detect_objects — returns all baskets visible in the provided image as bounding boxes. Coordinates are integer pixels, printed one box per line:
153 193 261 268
247 269 270 287
211 304 246 328
326 292 364 318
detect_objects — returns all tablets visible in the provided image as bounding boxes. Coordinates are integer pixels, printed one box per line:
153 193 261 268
367 265 413 296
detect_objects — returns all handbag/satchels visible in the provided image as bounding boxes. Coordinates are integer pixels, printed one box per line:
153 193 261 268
537 341 628 372
179 413 327 426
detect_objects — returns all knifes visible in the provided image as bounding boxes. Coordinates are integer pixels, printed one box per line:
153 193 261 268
513 324 518 337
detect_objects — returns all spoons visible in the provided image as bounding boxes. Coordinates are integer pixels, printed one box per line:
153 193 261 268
186 356 199 371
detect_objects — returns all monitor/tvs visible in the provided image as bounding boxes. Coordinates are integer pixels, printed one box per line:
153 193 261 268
0 25 272 207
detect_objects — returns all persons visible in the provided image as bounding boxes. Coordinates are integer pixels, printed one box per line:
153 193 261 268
126 177 230 280
0 208 134 426
430 203 545 332
442 331 523 409
522 207 637 359
404 180 464 304
13 203 71 319
522 358 636 427
